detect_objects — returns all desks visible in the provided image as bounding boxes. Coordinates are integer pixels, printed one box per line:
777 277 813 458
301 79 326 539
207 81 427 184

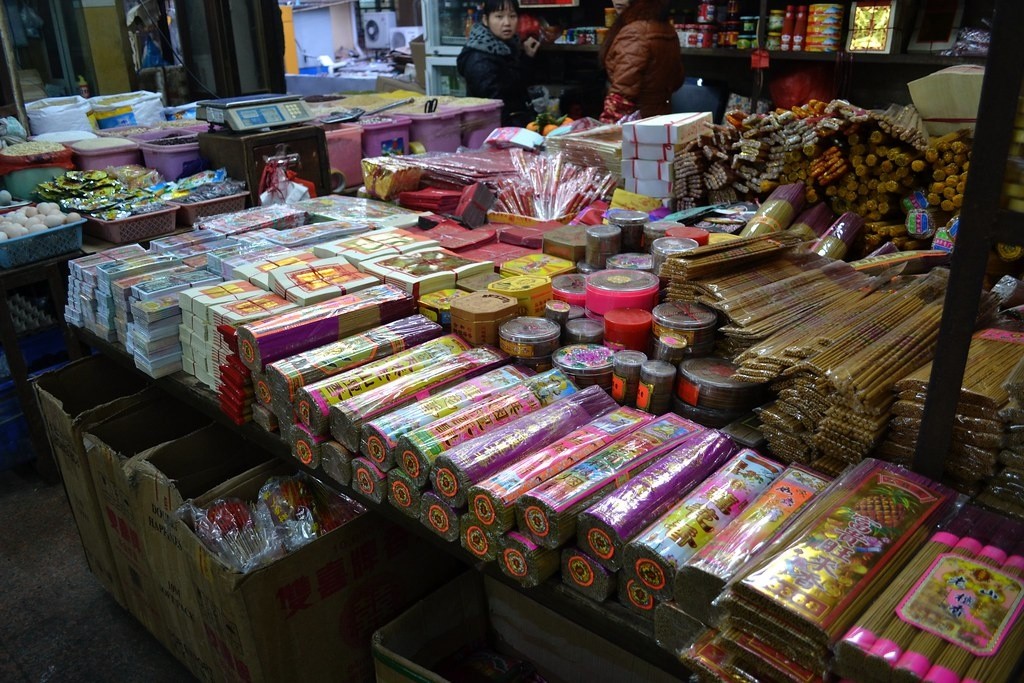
83 224 194 250
199 123 333 205
286 73 393 97
63 323 721 683
0 250 84 397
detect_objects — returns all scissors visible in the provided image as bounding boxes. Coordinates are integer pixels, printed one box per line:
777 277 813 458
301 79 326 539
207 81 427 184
424 98 438 114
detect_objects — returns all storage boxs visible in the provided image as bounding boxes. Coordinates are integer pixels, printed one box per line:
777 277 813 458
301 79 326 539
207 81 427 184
409 34 426 90
351 114 412 157
186 124 221 131
96 125 152 137
128 128 198 142
372 568 683 683
319 122 364 189
394 105 465 154
0 328 465 683
139 134 204 182
465 99 505 150
68 138 141 170
375 77 426 95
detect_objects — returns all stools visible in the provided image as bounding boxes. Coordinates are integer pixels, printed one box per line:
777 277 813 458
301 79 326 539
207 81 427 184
18 396 60 487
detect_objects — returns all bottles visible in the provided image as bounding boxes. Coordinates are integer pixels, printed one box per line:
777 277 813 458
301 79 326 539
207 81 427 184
441 0 483 97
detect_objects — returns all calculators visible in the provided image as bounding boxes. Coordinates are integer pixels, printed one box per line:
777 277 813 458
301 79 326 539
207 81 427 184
319 106 365 125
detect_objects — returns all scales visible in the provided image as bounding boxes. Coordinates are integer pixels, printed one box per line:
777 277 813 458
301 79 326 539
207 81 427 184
194 92 317 132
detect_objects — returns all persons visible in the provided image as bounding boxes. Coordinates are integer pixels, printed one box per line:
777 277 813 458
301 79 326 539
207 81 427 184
598 0 685 125
457 0 541 128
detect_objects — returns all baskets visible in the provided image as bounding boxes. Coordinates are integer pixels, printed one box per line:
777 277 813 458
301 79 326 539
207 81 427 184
82 201 180 244
0 203 88 270
171 190 252 227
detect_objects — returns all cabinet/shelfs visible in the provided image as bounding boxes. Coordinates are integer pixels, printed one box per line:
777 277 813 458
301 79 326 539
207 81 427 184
521 0 987 117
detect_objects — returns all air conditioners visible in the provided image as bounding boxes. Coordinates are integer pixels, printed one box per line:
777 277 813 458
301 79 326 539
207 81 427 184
364 12 397 49
390 26 423 50
